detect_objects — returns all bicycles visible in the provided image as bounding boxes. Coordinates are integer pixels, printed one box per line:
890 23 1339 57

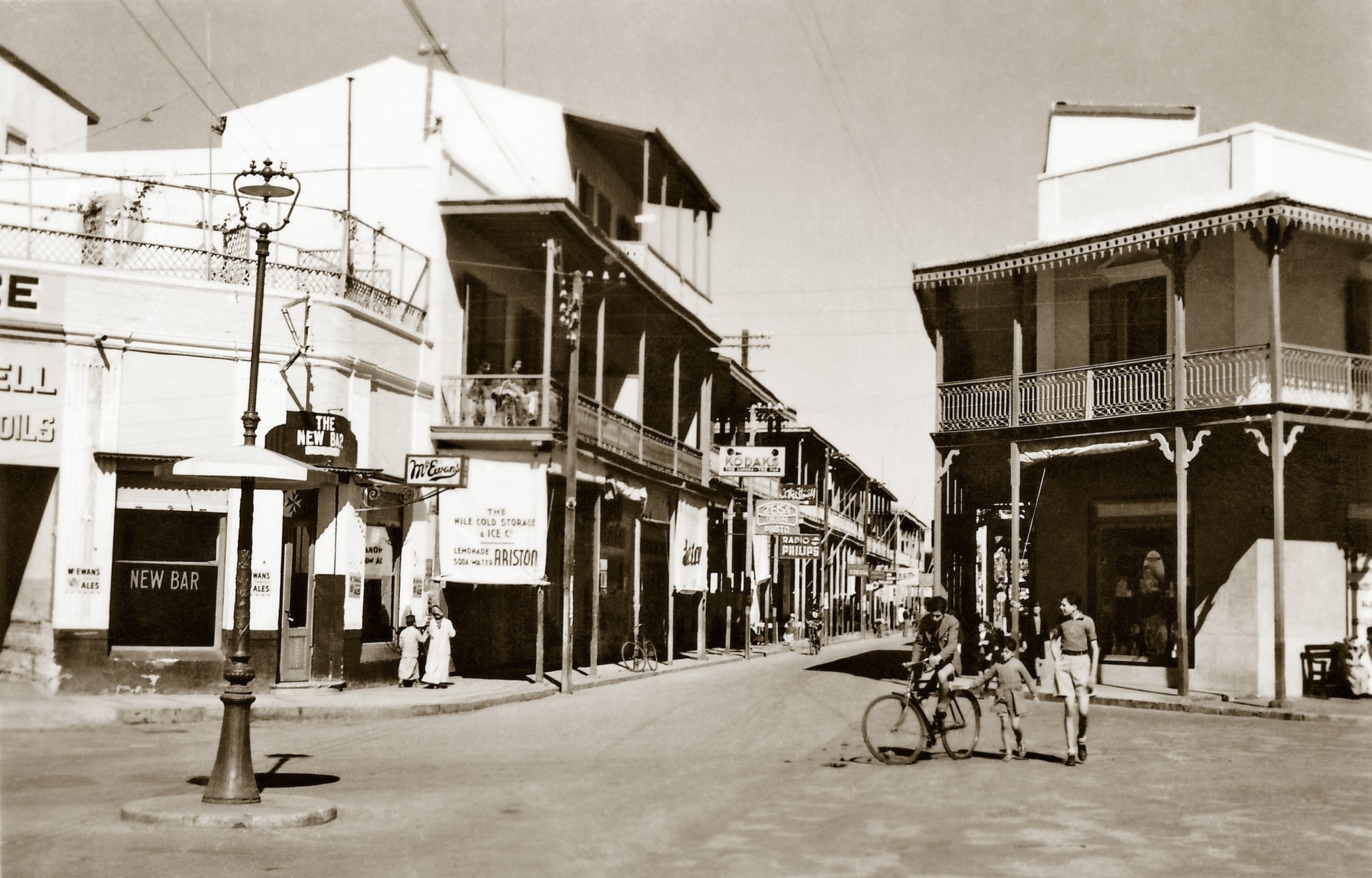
808 623 820 655
862 657 981 764
621 624 658 672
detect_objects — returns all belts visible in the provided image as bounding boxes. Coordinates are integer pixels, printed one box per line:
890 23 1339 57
1061 649 1088 655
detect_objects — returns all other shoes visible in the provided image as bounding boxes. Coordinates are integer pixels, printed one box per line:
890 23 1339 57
397 681 405 688
1017 741 1027 758
1002 752 1013 761
1077 739 1086 761
1065 753 1077 766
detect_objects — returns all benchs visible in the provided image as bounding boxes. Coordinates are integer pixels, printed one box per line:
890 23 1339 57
1305 642 1351 699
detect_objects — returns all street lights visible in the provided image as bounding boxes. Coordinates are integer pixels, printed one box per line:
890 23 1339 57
201 158 302 803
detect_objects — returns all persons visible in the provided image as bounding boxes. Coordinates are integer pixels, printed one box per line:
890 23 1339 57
806 604 824 648
468 358 558 426
1021 604 1045 686
782 613 795 651
903 606 909 637
966 636 1039 762
398 615 429 688
421 605 456 689
911 595 962 748
972 621 995 698
1051 591 1099 766
